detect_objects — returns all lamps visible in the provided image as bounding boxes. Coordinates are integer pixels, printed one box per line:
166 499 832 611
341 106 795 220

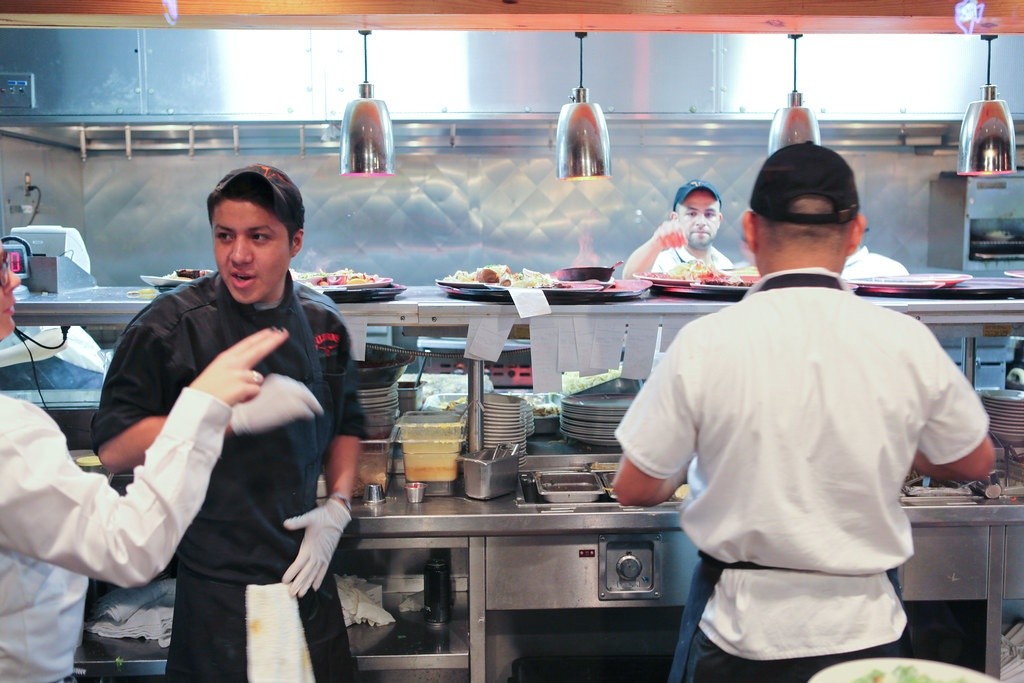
768 34 821 160
341 30 391 178
555 33 610 182
957 35 1016 175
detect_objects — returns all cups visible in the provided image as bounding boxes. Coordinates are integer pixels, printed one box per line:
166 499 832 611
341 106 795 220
366 484 386 506
405 482 427 504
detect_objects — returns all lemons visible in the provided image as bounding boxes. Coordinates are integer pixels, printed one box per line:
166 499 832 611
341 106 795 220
346 279 366 284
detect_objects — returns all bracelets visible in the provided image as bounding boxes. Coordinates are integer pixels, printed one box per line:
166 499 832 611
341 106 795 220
334 493 352 513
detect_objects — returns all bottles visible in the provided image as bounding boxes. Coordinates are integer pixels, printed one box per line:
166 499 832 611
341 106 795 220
422 558 449 626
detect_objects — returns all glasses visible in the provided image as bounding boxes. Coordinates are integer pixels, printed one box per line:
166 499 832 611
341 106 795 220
0 252 10 289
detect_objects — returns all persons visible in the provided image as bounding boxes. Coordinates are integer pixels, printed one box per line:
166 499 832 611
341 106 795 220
841 245 910 285
88 164 366 683
0 238 290 683
612 140 996 683
622 179 734 280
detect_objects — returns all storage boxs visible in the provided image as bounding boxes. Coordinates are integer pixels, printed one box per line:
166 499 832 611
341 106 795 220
398 410 466 481
350 424 401 499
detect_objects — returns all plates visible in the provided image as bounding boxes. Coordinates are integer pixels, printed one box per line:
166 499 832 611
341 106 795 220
482 392 535 467
537 283 604 292
605 279 654 293
633 272 694 286
846 273 974 289
319 278 394 289
690 282 750 294
139 274 189 286
435 279 492 289
559 391 636 447
981 389 1024 445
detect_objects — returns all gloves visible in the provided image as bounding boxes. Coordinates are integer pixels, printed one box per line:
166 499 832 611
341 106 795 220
284 498 352 599
228 370 325 436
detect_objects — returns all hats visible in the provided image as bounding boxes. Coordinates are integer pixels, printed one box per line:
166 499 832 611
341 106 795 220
749 140 860 225
673 181 721 216
208 165 305 234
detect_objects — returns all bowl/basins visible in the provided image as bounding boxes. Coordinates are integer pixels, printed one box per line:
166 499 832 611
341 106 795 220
556 266 616 283
354 344 416 440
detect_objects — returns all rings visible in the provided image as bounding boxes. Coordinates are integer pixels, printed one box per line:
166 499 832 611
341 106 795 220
252 370 259 383
